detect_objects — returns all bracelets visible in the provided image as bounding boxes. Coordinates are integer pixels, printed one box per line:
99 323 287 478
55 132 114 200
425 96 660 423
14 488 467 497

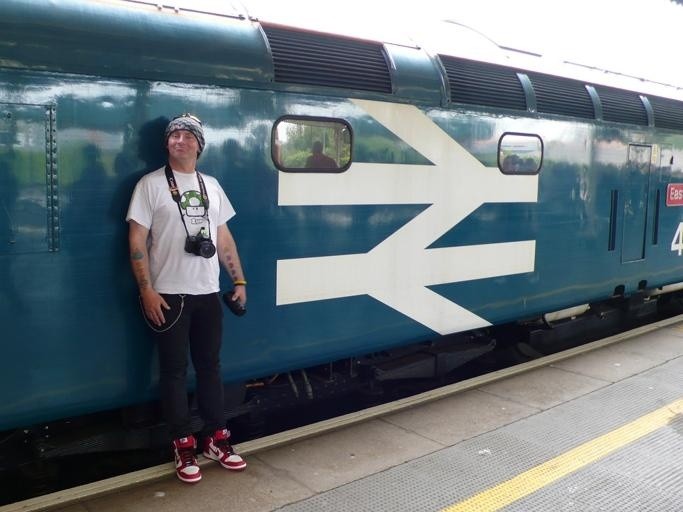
231 280 248 287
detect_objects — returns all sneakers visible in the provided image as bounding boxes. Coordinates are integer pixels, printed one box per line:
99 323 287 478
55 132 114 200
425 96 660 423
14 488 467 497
172 436 202 482
203 429 247 470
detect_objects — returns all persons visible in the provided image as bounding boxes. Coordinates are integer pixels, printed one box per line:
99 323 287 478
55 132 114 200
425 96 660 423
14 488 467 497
304 140 340 169
125 113 250 483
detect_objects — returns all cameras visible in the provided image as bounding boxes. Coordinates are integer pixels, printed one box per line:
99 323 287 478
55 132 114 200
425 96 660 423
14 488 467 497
184 227 216 258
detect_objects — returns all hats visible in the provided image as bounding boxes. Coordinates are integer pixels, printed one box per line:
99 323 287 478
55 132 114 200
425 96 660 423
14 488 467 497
164 117 205 158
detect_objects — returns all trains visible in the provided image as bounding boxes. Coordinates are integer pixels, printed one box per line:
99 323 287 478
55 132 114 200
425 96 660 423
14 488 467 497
0 0 682 462
